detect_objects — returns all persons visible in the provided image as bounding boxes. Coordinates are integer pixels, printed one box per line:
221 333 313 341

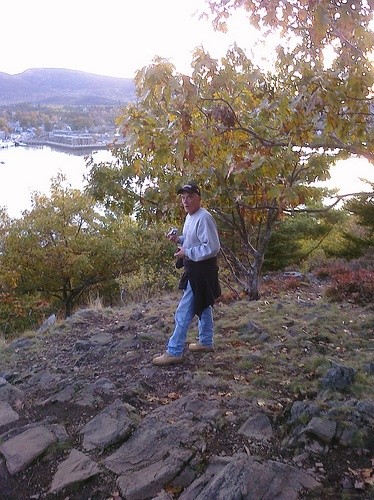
153 184 220 365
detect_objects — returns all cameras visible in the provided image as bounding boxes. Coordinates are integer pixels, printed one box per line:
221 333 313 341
169 229 178 234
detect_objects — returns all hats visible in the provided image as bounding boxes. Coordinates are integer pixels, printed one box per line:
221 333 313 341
177 184 200 196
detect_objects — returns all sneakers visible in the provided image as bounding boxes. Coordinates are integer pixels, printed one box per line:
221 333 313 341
189 344 213 351
153 353 183 365
